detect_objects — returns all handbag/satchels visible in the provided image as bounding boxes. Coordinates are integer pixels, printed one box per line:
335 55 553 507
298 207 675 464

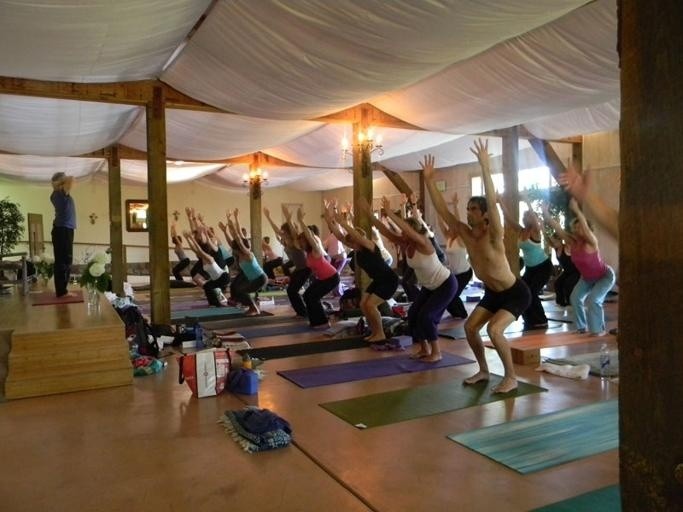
103 290 264 404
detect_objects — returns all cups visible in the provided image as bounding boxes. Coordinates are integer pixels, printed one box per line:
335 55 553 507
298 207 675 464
174 324 185 334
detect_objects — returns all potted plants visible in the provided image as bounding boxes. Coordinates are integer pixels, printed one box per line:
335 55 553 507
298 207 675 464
0 195 26 290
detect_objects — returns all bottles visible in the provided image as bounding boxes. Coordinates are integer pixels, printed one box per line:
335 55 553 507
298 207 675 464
600 343 610 379
194 323 207 349
600 380 609 401
241 352 252 369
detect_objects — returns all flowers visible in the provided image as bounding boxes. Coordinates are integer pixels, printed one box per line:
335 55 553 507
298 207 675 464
76 252 113 293
31 253 55 285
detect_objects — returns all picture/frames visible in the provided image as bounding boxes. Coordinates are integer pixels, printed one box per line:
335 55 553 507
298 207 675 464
125 199 150 233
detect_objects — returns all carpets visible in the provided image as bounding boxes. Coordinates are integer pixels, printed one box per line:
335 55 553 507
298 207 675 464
29 290 85 307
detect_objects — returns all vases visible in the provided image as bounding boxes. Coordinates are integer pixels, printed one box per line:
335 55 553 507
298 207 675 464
87 289 100 316
41 273 48 290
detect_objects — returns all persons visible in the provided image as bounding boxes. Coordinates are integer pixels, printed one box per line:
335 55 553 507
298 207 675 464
558 158 620 242
416 135 535 395
168 192 621 365
47 171 79 298
